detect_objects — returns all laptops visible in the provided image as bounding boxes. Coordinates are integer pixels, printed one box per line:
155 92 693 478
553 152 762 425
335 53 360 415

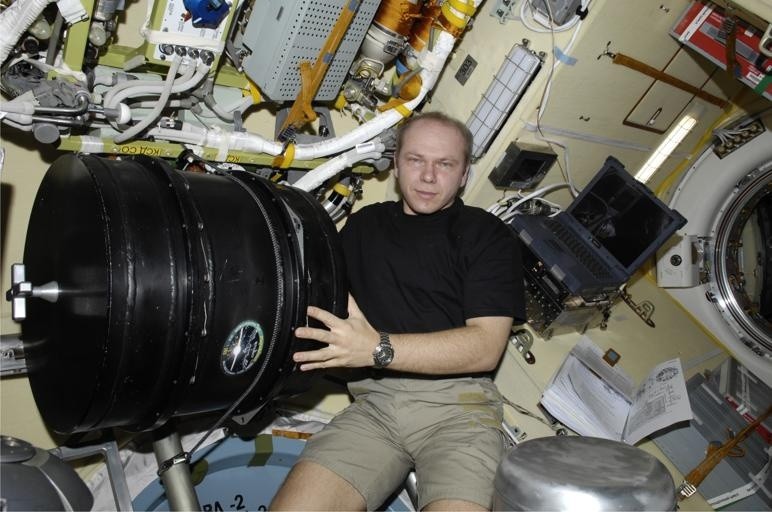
510 156 688 296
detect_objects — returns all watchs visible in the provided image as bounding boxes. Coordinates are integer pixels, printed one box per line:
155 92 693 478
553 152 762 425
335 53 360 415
373 331 394 369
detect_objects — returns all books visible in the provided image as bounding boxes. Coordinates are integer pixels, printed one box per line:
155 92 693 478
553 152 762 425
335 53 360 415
539 334 638 443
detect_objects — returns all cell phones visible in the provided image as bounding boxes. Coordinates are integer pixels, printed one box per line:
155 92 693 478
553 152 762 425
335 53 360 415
603 347 621 367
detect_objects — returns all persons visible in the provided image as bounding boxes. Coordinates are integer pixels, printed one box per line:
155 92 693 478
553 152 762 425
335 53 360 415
266 113 527 512
